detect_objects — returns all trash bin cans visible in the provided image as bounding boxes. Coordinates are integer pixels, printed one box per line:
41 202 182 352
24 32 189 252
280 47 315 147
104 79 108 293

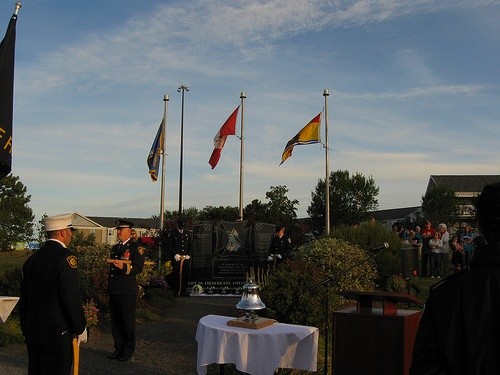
330 290 424 375
399 247 419 277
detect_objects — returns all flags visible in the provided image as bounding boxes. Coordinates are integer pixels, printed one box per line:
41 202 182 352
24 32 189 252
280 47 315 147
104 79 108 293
0 14 17 180
147 114 166 182
279 111 323 164
209 105 240 169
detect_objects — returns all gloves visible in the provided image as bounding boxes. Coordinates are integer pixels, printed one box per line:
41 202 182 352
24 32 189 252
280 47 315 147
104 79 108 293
185 255 190 260
277 254 282 259
174 254 181 261
77 326 88 346
270 254 274 256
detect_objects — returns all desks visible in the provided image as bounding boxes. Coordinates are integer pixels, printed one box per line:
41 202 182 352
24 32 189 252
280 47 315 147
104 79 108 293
195 314 319 375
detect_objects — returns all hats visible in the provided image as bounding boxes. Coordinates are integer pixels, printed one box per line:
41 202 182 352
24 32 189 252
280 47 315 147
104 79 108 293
42 213 77 232
275 225 283 231
114 219 134 230
472 182 500 218
177 218 185 226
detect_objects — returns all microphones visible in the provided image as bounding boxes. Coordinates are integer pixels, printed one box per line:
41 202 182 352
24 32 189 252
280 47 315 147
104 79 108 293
371 242 390 251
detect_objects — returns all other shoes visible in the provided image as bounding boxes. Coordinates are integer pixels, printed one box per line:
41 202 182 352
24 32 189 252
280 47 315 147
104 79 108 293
431 265 470 280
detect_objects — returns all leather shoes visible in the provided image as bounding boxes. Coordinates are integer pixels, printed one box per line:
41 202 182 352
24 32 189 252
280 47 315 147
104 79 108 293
108 351 118 359
118 356 128 361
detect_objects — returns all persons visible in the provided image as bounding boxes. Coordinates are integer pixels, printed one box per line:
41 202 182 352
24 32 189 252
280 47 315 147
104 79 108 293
268 224 292 265
19 213 87 375
168 218 193 297
392 217 479 279
408 181 500 375
131 229 143 244
107 219 145 362
